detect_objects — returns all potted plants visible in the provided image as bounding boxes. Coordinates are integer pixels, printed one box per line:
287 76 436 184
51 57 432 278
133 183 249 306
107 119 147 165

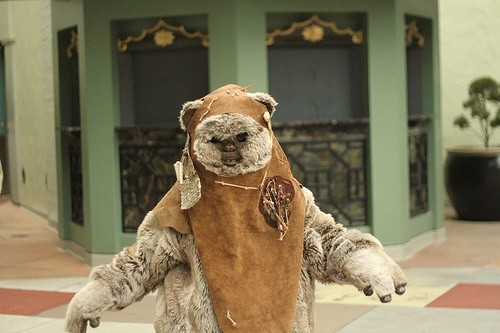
443 77 500 222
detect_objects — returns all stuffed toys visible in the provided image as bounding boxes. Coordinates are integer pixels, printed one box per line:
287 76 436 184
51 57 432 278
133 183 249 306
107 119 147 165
65 84 408 333
